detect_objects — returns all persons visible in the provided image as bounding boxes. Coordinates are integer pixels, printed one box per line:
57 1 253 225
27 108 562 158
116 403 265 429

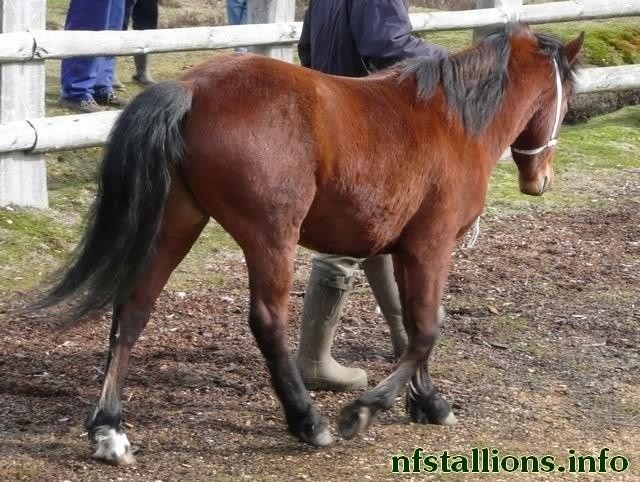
228 0 250 53
110 0 158 90
54 0 130 114
294 0 456 394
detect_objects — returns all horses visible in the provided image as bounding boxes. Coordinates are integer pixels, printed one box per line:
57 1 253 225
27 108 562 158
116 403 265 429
6 12 587 468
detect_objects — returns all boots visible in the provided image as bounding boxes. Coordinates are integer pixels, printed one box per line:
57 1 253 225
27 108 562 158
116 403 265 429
131 54 158 89
360 254 447 360
294 262 369 392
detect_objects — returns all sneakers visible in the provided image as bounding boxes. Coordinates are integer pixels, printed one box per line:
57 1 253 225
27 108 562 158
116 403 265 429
57 96 105 114
93 93 129 109
110 71 126 91
